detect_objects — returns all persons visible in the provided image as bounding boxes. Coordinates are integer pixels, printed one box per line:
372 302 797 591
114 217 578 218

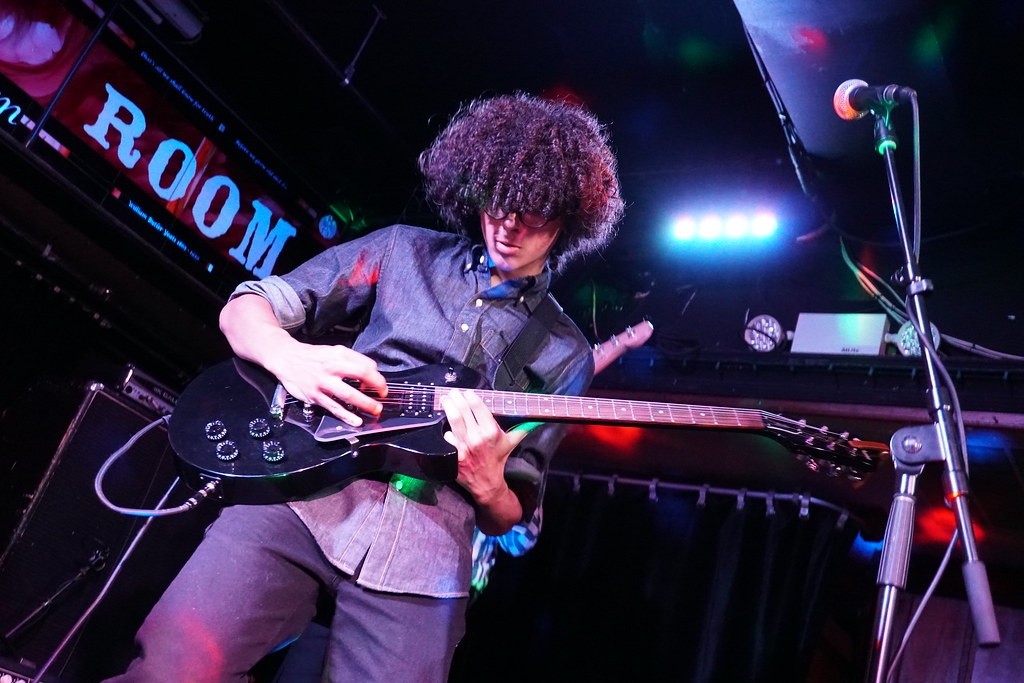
98 93 628 682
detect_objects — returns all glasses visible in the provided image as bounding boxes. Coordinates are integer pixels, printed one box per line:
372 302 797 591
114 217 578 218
483 201 559 228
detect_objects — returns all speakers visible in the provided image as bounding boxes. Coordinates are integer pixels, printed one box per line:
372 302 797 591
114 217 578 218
0 381 222 683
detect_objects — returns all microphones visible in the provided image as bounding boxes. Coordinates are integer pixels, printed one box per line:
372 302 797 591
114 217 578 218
92 549 105 571
833 78 918 120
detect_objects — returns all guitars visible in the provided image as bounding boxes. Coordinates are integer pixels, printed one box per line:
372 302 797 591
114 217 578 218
166 355 890 506
